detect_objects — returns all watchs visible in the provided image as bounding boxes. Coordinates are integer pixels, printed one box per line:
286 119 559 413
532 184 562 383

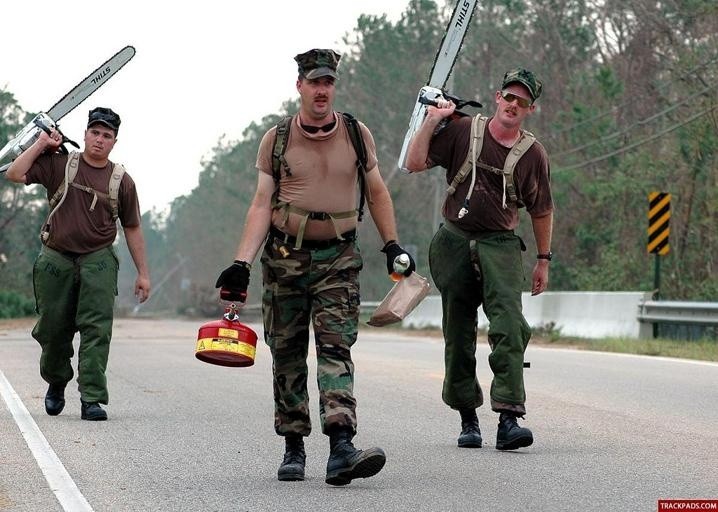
536 251 553 262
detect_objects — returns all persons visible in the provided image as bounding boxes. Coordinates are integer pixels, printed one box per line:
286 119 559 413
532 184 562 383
406 63 556 451
215 48 416 487
5 105 152 421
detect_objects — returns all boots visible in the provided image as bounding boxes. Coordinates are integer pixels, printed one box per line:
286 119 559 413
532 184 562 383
457 409 483 448
278 433 307 481
496 412 533 450
325 429 386 487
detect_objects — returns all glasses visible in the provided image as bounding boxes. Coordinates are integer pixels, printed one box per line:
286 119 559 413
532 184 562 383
500 90 532 109
91 112 115 125
300 120 337 134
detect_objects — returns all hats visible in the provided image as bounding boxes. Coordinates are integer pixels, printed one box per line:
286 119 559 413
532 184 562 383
87 107 120 130
294 49 341 81
502 66 543 100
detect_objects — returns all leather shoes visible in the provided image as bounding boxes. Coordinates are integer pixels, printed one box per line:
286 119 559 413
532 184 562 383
45 384 65 415
81 401 107 420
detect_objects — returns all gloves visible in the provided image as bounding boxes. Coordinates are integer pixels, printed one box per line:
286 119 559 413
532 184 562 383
380 239 416 278
216 259 251 309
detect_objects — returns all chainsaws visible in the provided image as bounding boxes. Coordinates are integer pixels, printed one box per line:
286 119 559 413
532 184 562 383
397 0 483 174
1 45 136 171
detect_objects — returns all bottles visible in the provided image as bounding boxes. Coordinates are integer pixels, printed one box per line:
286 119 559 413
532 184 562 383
388 254 412 282
